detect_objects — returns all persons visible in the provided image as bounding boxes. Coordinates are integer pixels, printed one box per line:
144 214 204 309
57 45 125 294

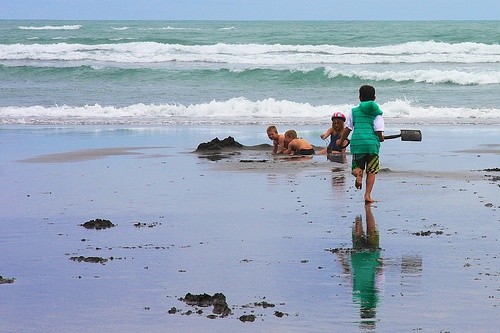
318 111 351 164
335 84 385 203
282 129 316 156
267 125 290 155
331 202 385 330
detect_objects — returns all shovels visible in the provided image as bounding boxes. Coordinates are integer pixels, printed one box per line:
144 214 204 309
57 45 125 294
335 129 423 151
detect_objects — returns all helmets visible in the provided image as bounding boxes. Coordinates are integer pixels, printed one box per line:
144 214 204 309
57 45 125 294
332 112 345 121
